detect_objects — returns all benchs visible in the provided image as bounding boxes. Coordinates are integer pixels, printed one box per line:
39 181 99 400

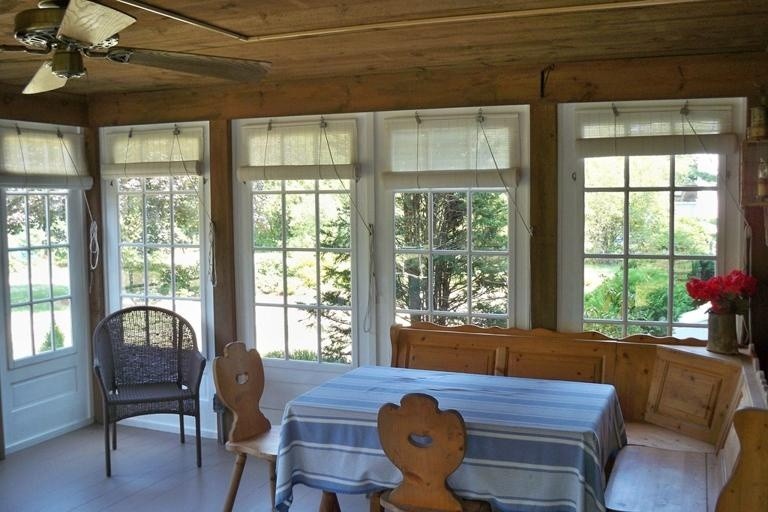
388 321 766 512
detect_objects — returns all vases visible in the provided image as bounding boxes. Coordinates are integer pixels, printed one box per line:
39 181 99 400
708 312 740 354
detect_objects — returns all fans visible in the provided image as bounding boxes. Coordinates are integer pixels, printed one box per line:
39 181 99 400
0 1 274 95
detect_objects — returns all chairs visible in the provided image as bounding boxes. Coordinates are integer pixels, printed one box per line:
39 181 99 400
374 393 488 512
92 306 206 478
212 342 282 511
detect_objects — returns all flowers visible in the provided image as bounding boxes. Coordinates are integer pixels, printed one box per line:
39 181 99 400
685 270 755 312
